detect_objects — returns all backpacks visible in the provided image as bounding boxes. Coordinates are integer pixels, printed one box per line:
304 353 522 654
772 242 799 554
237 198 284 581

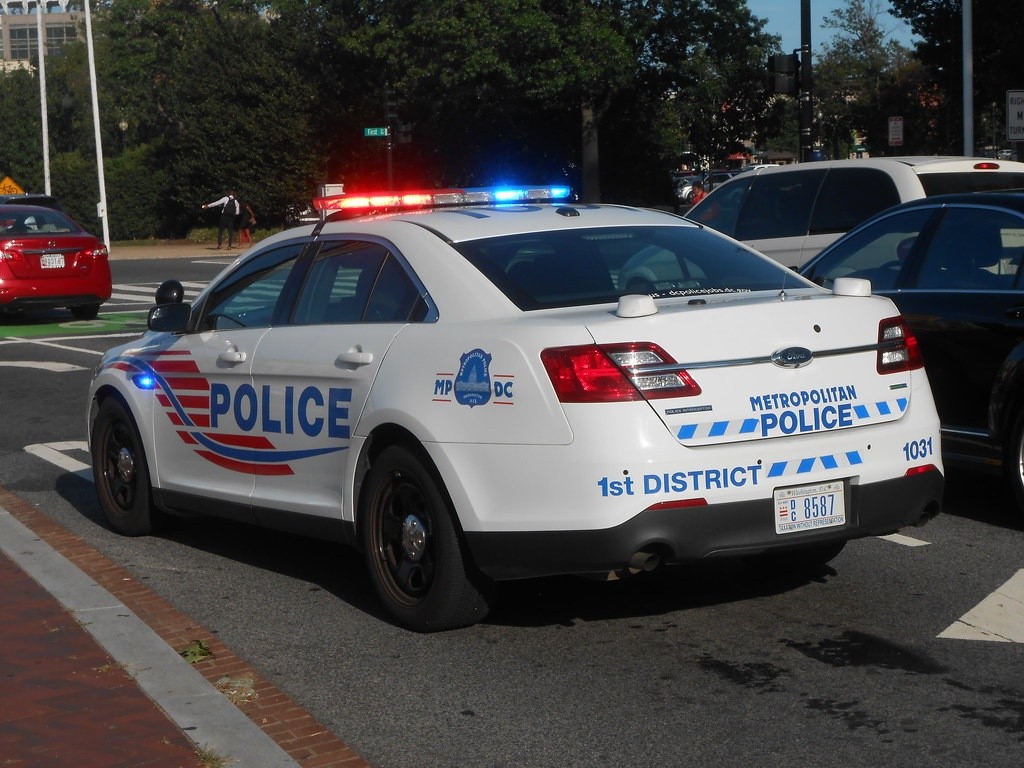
223 196 236 217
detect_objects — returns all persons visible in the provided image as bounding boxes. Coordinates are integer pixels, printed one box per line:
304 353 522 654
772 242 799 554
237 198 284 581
689 181 720 227
237 197 256 246
201 191 240 250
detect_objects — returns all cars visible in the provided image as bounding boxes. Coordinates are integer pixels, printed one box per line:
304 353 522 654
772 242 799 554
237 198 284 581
671 163 784 206
617 153 1024 300
0 193 113 320
79 181 950 634
789 188 1024 516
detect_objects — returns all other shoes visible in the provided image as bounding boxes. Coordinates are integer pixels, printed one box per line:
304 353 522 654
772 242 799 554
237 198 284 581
218 245 222 248
237 244 244 249
228 246 231 251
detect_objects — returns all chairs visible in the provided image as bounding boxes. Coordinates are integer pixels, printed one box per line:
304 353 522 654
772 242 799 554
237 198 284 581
948 214 1004 290
350 257 394 322
